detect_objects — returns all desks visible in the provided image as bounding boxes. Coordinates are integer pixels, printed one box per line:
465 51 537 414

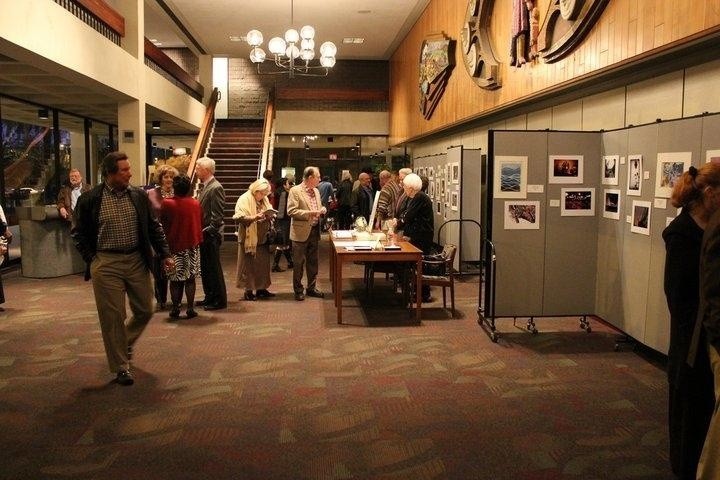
328 230 424 323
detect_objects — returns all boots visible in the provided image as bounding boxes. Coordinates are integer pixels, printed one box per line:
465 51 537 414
283 248 294 268
271 249 286 272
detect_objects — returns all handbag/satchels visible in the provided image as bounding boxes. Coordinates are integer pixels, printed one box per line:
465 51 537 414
424 254 446 275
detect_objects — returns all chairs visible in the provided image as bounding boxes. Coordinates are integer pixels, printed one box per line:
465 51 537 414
405 244 458 314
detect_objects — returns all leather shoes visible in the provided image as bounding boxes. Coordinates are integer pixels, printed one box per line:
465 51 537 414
196 299 226 311
295 293 305 301
307 288 323 298
118 347 134 386
256 290 275 297
186 309 199 318
245 292 256 300
157 301 180 318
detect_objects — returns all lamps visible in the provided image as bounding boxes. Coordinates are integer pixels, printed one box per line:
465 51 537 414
247 0 338 79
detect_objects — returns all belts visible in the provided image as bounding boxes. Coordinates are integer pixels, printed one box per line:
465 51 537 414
104 247 140 255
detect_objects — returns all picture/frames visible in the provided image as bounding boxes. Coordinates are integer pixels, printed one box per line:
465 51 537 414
494 156 529 200
601 155 619 185
413 160 461 220
655 151 690 201
504 202 540 230
626 155 644 196
630 199 651 236
548 155 585 185
560 188 595 216
603 188 621 220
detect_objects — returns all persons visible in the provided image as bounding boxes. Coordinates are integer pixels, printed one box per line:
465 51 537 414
0 202 15 312
70 152 176 388
233 166 436 302
57 156 229 317
662 160 720 480
688 208 719 480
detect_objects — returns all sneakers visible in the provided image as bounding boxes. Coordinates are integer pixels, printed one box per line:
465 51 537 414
413 295 432 304
